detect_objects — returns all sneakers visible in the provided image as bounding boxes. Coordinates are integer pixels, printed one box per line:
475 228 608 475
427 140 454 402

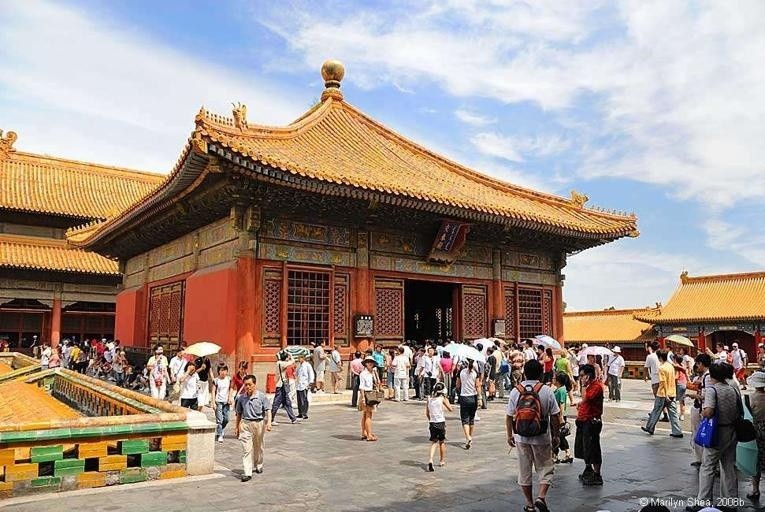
312 387 343 394
686 504 737 512
680 415 684 421
579 469 603 485
217 430 226 443
439 462 444 466
385 396 424 402
608 398 619 402
670 434 683 438
256 468 262 473
292 414 308 423
741 387 746 390
466 437 471 449
660 418 669 422
641 427 653 435
429 461 435 471
271 421 279 426
242 476 250 481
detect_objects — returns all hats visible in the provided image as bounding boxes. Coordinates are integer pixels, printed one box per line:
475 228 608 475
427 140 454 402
433 382 447 394
361 356 378 367
746 372 765 388
732 343 738 347
611 346 621 352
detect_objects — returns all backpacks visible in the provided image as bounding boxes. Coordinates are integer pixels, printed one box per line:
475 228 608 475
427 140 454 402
512 382 548 437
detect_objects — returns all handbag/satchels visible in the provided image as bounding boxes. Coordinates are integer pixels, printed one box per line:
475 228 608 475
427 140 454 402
736 419 756 442
559 428 570 436
171 376 177 383
694 412 720 448
364 391 381 406
501 359 508 373
282 382 290 393
739 349 749 364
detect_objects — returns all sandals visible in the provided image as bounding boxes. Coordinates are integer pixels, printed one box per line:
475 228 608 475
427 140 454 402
535 497 550 512
361 434 377 441
524 505 536 512
746 491 760 499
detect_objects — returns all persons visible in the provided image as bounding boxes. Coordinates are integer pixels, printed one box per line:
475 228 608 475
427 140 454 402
26 336 765 510
0 339 11 352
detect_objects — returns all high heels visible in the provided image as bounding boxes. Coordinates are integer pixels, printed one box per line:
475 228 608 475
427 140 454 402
552 454 573 463
